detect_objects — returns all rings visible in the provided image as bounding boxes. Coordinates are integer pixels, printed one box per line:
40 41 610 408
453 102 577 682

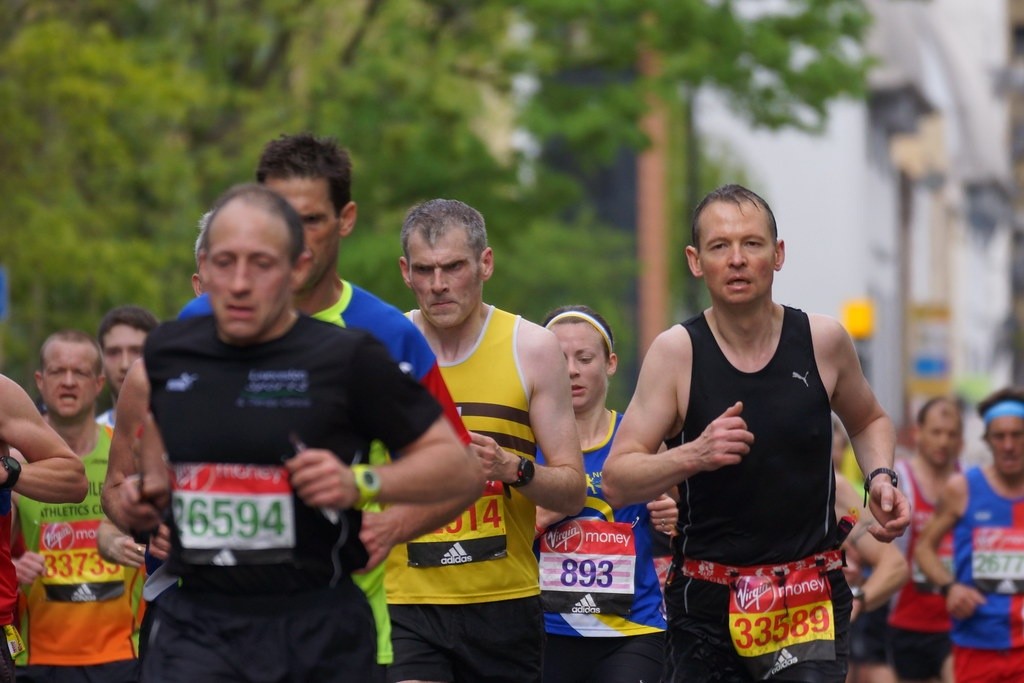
662 518 665 527
137 546 141 554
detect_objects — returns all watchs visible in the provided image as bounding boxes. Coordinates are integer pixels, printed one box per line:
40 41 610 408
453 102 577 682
0 456 21 489
863 468 898 508
503 455 535 499
351 463 381 510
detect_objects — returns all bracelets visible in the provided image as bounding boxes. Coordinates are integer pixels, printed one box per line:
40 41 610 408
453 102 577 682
852 588 865 602
940 580 954 596
535 525 545 539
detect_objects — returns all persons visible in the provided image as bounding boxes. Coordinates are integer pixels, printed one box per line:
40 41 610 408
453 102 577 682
385 196 586 683
96 132 486 683
531 305 679 683
0 374 89 683
94 308 160 428
13 328 149 683
101 189 475 683
832 388 1024 683
602 180 911 683
